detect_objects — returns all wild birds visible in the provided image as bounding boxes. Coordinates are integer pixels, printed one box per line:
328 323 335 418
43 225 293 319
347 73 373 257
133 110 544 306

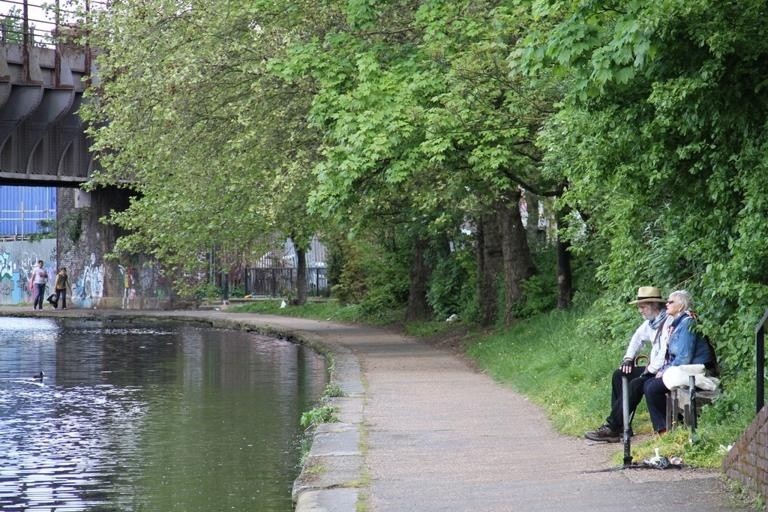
644 446 669 472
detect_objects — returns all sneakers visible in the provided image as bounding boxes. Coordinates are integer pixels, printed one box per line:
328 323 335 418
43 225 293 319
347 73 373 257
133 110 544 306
585 425 620 443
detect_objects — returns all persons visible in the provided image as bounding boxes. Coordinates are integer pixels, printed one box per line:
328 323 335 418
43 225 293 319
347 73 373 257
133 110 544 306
78 250 105 310
118 261 131 309
583 285 676 443
127 270 141 301
30 260 49 311
51 268 73 311
641 289 721 439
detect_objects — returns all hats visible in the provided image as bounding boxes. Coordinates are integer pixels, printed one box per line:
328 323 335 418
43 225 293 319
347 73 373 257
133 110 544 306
629 287 668 305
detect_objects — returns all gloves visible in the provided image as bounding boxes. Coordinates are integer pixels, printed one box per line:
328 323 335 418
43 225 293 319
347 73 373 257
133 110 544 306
620 360 634 366
